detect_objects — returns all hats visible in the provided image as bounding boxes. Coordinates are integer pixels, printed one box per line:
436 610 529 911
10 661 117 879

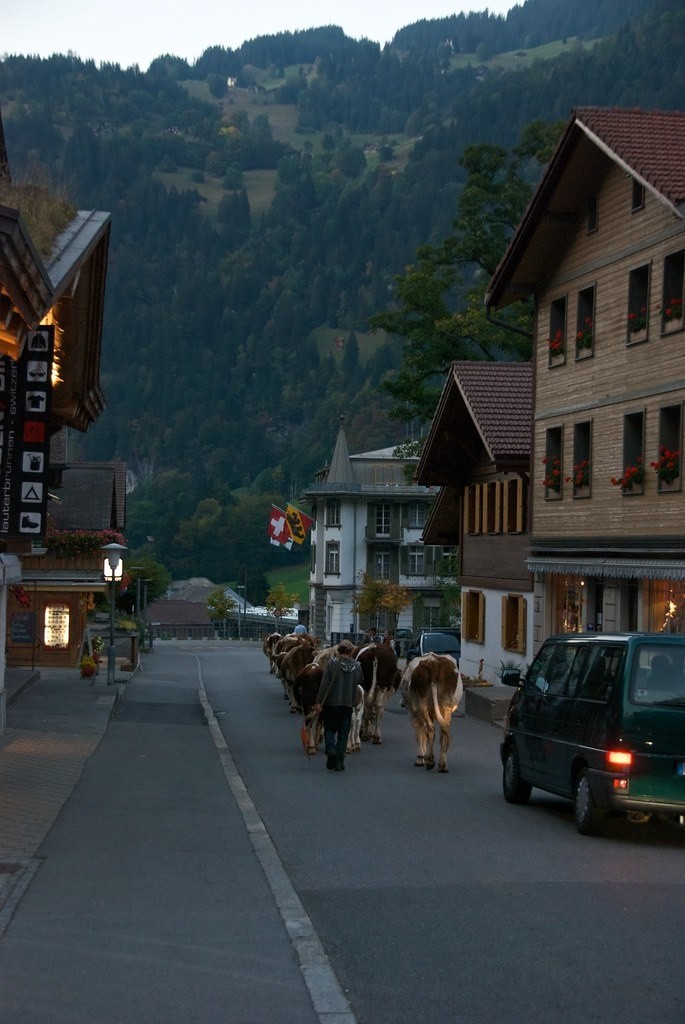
338 639 356 650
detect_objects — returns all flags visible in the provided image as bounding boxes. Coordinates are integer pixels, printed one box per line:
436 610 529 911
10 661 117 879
270 536 293 551
282 506 312 545
267 506 288 544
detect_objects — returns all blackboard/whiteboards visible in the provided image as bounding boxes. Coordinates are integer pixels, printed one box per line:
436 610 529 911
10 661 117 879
9 610 37 643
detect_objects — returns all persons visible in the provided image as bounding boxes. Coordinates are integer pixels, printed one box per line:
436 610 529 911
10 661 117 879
294 621 307 634
383 631 398 658
362 627 381 644
314 640 364 771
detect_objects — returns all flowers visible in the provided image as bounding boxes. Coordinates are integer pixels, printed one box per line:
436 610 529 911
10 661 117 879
659 297 682 321
544 327 565 360
627 307 647 334
45 529 129 562
649 442 681 485
541 454 561 494
609 456 643 493
564 458 591 488
574 316 593 349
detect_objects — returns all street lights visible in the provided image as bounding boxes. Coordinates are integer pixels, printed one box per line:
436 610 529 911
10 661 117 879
237 585 246 637
141 578 153 645
99 543 128 687
131 566 146 648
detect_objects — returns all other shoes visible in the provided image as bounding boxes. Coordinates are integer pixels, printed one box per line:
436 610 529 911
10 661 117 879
327 753 337 770
335 761 344 771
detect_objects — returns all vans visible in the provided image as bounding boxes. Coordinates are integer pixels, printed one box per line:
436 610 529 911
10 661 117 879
500 631 685 836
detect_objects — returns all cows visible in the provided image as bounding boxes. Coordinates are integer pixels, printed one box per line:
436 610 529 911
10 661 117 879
263 632 404 755
401 652 464 773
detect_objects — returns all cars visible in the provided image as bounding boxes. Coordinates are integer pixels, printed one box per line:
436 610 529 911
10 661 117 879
393 627 412 651
406 631 460 672
